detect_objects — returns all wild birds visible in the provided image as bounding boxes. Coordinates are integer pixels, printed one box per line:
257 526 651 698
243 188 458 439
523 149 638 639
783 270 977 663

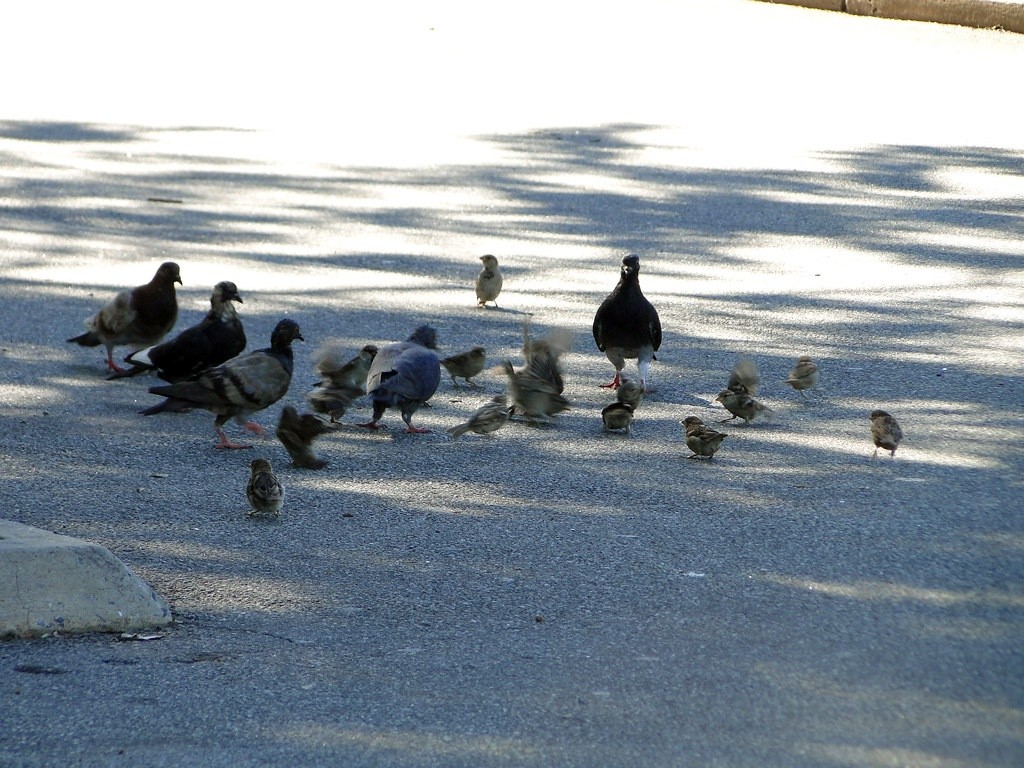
247 345 379 518
439 254 575 438
682 356 903 460
602 372 644 435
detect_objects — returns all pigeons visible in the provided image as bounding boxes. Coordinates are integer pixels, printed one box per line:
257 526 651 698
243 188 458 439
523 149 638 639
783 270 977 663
593 254 662 392
66 262 305 449
357 324 441 433
